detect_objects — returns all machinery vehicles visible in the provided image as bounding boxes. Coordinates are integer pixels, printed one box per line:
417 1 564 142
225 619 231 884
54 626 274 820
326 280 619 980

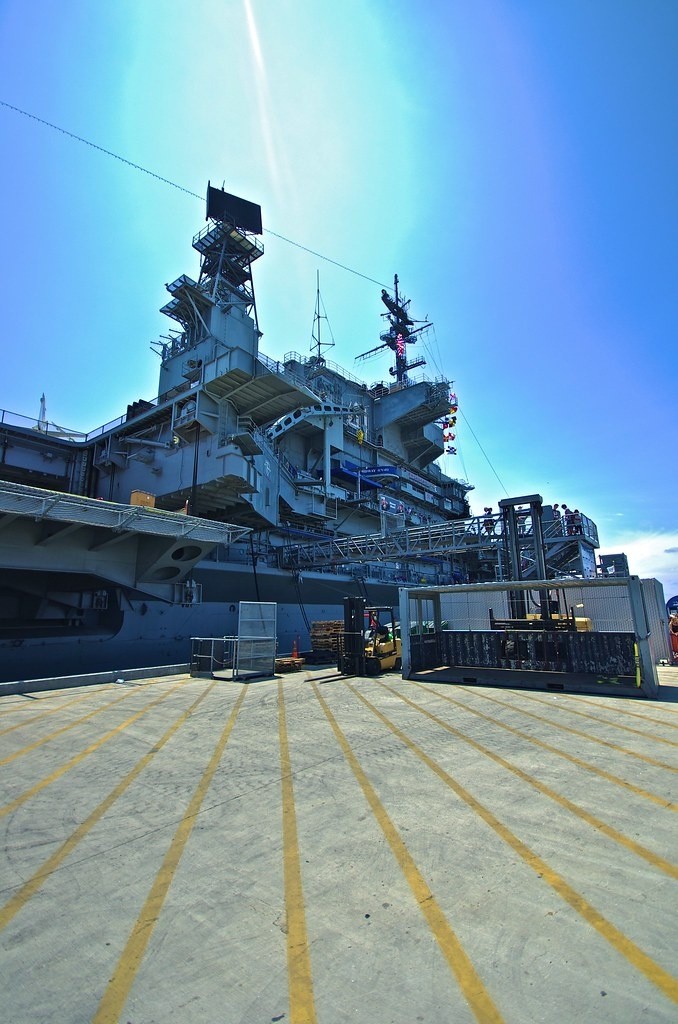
487 493 642 687
335 596 402 677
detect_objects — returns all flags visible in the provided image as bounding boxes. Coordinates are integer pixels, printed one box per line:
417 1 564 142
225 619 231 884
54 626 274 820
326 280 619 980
443 393 457 454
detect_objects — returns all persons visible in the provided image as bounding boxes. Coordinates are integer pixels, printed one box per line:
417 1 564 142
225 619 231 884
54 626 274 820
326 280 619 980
405 506 437 524
669 616 678 657
483 504 583 536
